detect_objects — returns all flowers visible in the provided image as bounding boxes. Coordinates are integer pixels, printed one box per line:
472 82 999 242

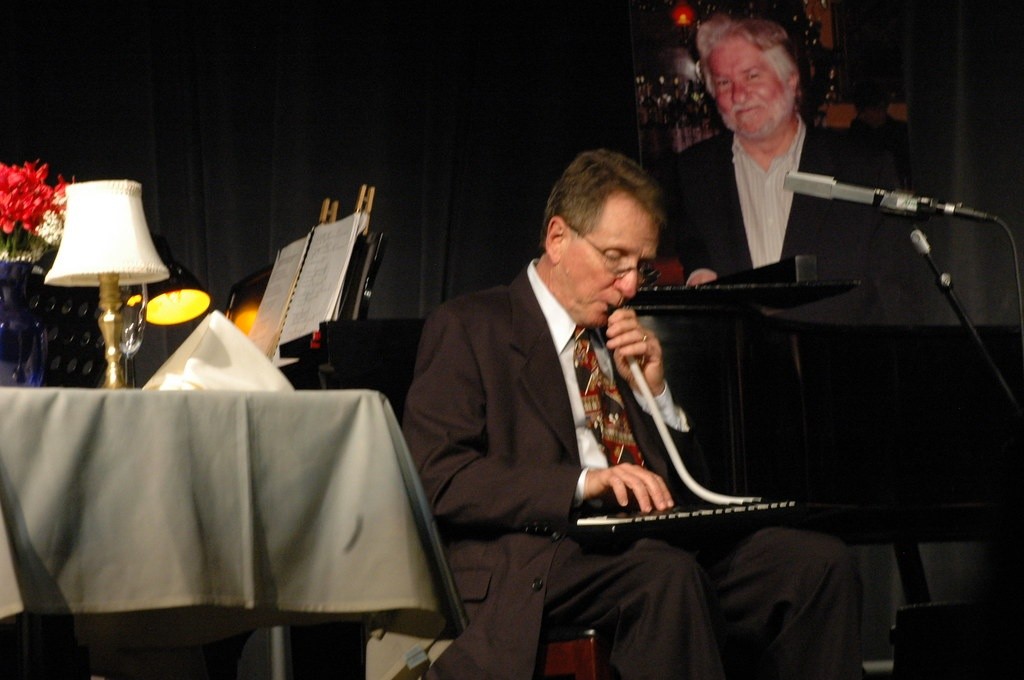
0 156 76 265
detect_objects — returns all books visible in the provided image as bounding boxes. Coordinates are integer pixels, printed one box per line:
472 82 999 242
247 210 361 365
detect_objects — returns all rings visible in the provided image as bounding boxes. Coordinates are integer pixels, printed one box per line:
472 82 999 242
643 333 648 341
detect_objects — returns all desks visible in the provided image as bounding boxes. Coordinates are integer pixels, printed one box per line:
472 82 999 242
0 387 467 680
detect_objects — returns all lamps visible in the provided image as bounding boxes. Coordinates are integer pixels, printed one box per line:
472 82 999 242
43 180 170 390
672 0 695 48
131 234 213 327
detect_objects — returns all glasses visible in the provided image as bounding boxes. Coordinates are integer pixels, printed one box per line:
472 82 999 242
566 224 656 284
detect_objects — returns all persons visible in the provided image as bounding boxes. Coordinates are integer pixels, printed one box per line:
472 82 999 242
667 12 900 288
401 150 862 680
848 97 912 189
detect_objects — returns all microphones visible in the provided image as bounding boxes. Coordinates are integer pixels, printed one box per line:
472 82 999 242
781 171 998 223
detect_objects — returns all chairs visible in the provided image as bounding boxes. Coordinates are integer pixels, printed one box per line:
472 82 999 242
318 317 619 680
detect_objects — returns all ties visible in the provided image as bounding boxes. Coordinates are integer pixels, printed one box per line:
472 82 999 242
572 325 646 506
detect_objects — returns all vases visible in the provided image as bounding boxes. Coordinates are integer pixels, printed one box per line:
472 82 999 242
0 260 46 389
